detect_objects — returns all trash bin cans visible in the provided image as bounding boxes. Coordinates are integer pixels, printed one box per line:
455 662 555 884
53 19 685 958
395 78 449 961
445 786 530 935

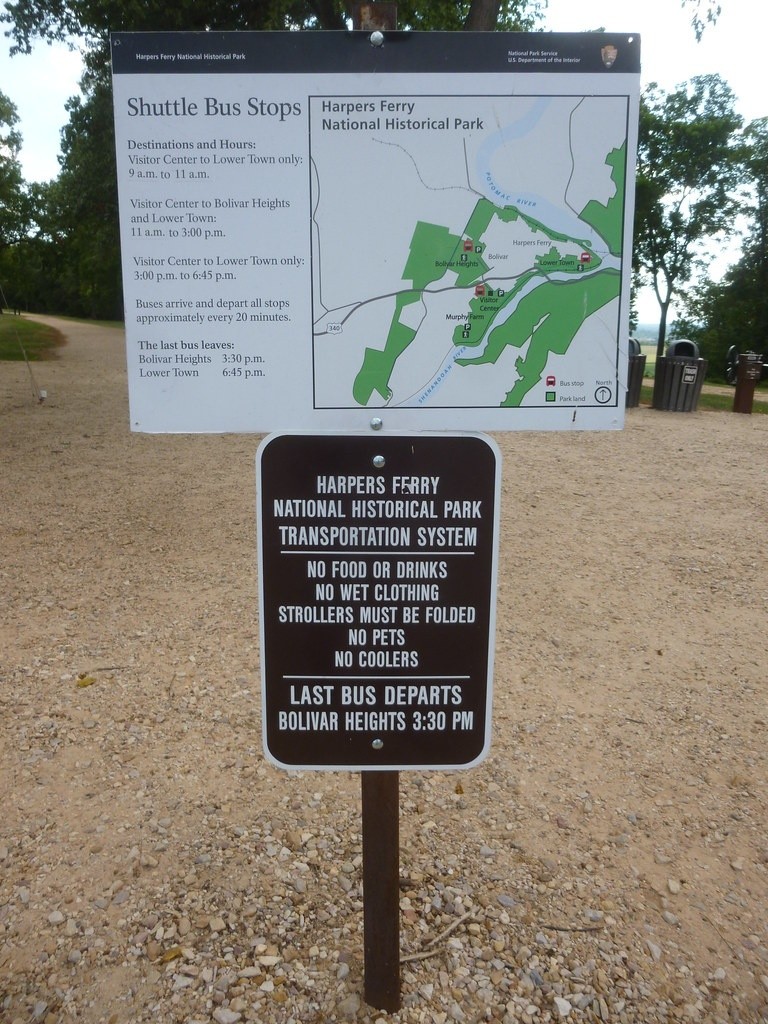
652 339 709 413
626 336 646 408
